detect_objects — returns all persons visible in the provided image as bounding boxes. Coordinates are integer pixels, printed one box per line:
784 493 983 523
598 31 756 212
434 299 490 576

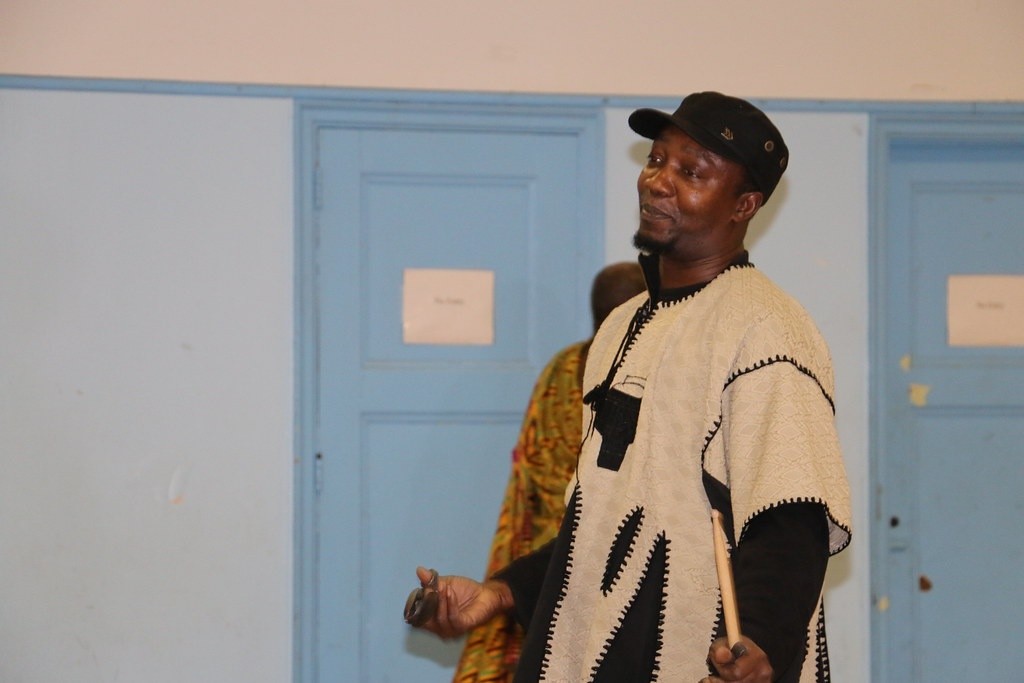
404 91 853 683
454 262 648 683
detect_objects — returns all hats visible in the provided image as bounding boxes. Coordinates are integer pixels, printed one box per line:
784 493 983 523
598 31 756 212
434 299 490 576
627 91 790 206
